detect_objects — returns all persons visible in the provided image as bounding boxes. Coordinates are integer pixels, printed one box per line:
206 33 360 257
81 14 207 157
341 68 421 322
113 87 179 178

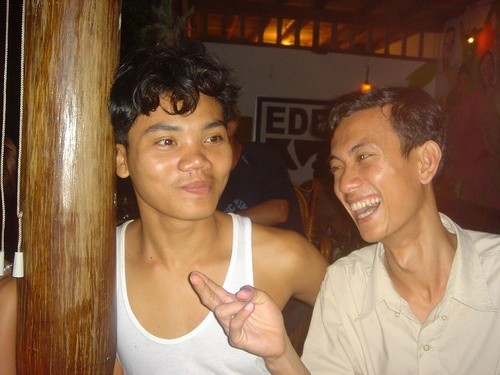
1 40 331 375
0 109 370 359
188 85 500 375
